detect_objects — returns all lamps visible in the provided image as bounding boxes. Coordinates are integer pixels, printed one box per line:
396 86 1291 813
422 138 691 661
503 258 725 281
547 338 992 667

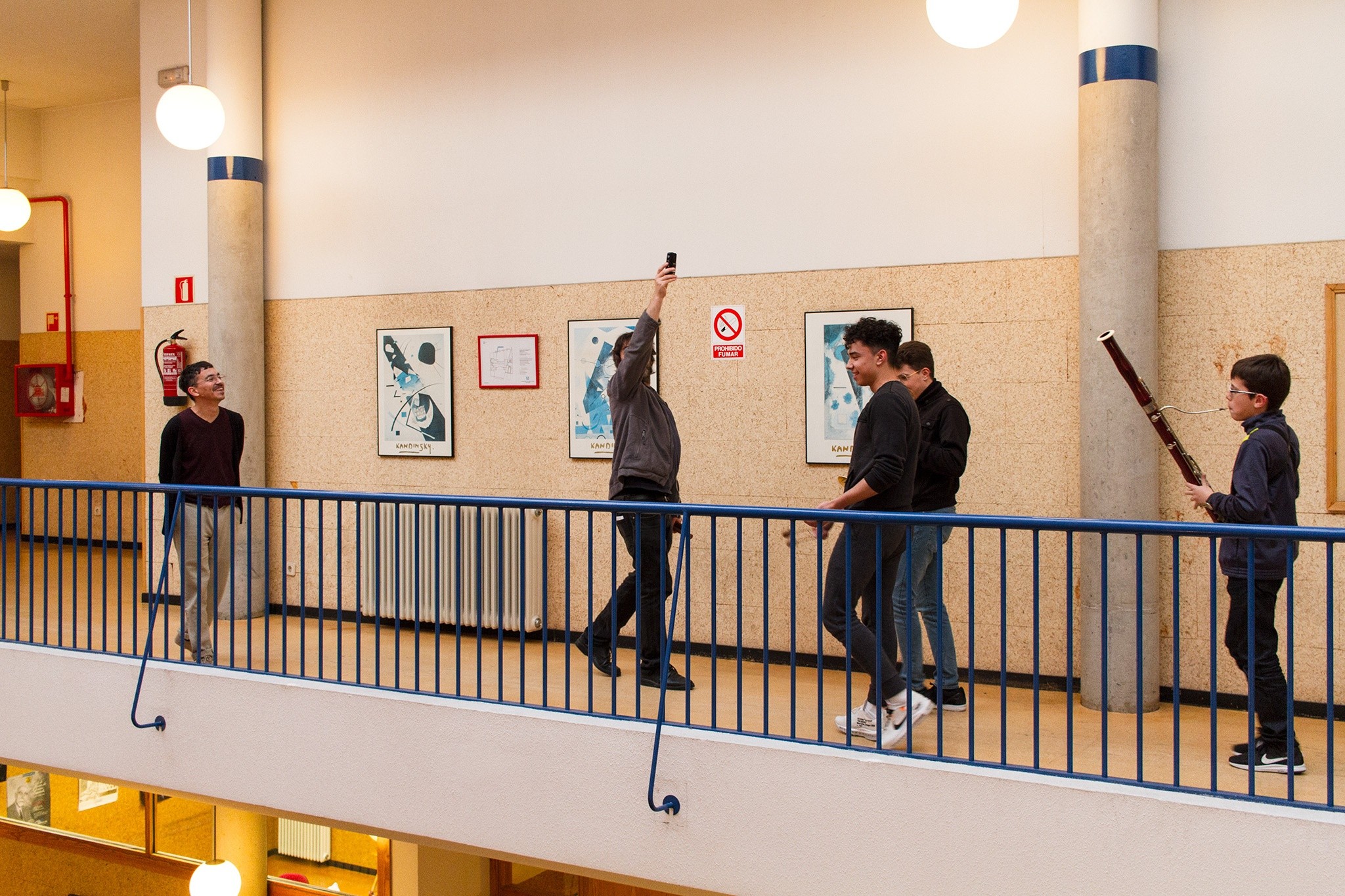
0 80 31 232
189 805 241 896
155 0 225 149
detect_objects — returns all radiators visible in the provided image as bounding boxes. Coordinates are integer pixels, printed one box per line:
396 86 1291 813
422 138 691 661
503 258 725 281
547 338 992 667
278 817 331 863
360 501 543 633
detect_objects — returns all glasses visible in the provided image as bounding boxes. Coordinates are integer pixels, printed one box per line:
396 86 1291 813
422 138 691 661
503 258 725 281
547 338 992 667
1228 384 1258 399
191 374 226 388
897 369 931 381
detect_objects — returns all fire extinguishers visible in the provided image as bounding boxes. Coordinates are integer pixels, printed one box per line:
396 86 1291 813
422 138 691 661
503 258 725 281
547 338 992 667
155 329 191 406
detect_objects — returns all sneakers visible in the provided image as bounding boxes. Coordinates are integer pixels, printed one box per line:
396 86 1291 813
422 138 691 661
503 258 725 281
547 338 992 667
877 697 934 749
1232 738 1267 754
1229 746 1307 773
919 686 968 711
175 631 192 650
191 640 214 665
836 699 883 741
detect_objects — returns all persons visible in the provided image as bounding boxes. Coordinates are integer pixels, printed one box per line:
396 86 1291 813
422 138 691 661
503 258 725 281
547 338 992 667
893 341 971 711
7 784 35 823
575 262 695 690
804 318 933 749
1184 353 1307 773
158 362 245 664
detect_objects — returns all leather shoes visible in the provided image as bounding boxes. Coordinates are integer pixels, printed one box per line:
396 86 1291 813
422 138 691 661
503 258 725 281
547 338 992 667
575 632 621 677
641 663 695 690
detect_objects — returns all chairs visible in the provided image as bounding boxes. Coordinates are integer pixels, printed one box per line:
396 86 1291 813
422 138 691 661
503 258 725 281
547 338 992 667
280 873 309 884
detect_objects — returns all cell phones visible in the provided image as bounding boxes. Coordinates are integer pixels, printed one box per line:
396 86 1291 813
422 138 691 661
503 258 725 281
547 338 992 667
666 253 677 274
